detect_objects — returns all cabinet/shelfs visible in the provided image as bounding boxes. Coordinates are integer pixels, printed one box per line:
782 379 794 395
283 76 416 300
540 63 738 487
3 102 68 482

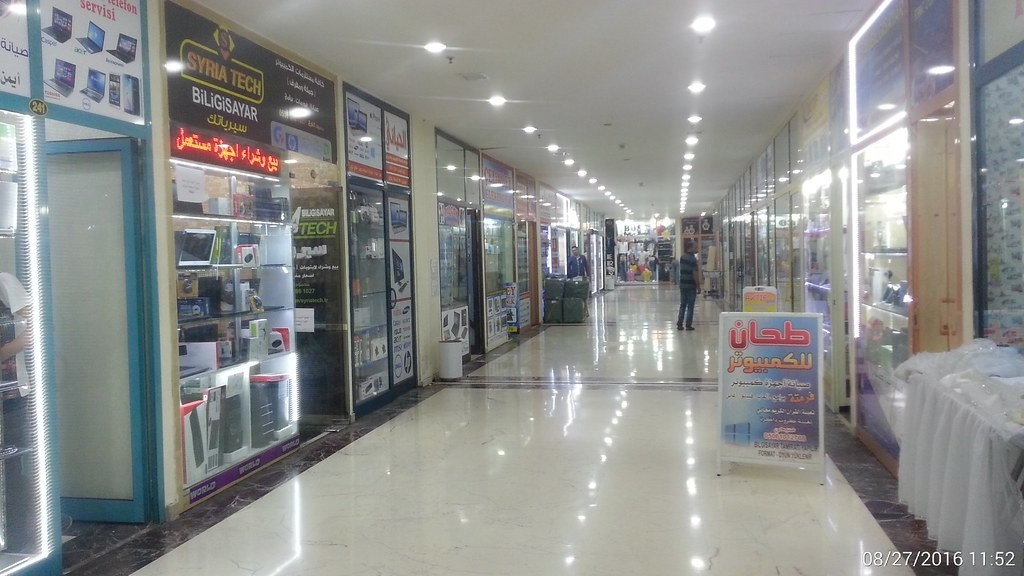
349 182 391 415
0 112 43 573
853 130 912 456
164 156 302 505
434 202 471 306
483 213 550 296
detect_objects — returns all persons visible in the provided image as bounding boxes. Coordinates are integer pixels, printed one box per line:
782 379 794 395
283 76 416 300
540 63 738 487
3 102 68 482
1 271 36 548
677 241 702 331
627 245 658 282
568 246 590 277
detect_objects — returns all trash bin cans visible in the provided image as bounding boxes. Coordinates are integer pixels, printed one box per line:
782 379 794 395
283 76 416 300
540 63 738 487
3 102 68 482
437 340 463 381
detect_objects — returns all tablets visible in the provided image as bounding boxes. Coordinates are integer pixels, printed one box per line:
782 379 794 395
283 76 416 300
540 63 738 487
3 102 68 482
108 73 120 108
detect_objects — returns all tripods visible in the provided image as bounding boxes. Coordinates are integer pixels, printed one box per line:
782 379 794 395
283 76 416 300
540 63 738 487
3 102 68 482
76 20 106 55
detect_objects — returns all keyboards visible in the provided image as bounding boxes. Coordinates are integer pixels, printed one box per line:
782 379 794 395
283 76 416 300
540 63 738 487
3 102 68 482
396 283 407 293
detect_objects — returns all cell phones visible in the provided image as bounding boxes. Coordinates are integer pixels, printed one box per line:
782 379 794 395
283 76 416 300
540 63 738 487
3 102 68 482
129 77 140 115
122 74 134 114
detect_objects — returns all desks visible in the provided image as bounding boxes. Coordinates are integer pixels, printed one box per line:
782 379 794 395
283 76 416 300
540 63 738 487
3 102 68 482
897 365 1024 576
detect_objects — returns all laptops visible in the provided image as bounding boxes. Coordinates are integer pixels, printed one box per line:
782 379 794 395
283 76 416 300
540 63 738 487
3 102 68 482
450 311 460 337
393 210 408 233
106 33 137 65
41 57 76 98
40 6 74 44
80 66 106 104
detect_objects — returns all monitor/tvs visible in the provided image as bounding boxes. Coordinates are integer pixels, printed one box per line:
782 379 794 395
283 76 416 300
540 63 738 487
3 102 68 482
390 202 401 222
176 229 217 267
347 98 359 125
354 111 368 132
443 314 448 328
392 249 404 283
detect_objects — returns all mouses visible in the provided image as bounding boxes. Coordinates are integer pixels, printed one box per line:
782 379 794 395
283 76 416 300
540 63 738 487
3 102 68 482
271 339 282 348
407 280 409 283
253 296 262 309
244 253 253 263
